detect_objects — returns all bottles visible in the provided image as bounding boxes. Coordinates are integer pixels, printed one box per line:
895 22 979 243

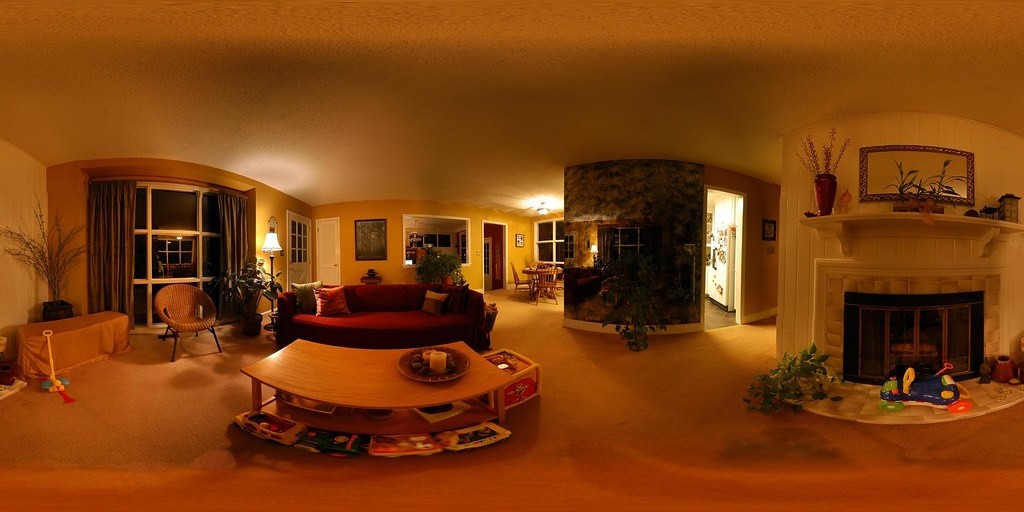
991 354 1017 383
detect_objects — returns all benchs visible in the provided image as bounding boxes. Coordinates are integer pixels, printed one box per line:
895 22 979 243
17 311 135 378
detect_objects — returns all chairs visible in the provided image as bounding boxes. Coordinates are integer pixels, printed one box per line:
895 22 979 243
154 284 222 362
511 261 558 306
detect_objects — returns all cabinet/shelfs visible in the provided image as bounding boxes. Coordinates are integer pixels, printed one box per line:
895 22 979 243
705 212 713 266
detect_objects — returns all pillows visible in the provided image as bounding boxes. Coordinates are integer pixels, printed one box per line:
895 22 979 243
291 281 351 317
422 284 469 315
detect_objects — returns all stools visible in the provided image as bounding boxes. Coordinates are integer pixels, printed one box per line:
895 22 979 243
481 348 542 410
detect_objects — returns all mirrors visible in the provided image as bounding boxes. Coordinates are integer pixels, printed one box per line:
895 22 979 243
859 146 975 206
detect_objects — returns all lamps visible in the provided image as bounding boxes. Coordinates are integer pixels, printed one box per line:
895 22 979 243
590 245 598 267
537 202 549 215
262 228 282 331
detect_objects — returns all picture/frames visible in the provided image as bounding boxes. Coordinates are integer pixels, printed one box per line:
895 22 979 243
516 234 524 247
355 219 387 261
762 219 776 241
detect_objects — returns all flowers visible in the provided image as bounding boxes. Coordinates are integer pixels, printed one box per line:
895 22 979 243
796 128 852 181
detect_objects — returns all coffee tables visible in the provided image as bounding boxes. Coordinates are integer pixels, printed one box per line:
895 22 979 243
240 338 516 434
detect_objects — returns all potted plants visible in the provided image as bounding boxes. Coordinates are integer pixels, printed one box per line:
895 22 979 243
208 256 283 336
883 157 974 213
415 248 467 287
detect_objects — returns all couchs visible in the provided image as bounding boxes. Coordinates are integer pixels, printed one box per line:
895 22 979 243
276 284 489 352
564 267 603 304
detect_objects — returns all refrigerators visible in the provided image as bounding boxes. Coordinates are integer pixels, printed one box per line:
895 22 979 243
708 227 736 312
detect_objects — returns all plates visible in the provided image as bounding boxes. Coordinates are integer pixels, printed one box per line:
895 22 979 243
416 403 454 415
397 346 471 382
364 409 393 420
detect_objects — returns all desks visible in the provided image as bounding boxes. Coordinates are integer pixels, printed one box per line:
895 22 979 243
522 268 563 299
361 276 382 284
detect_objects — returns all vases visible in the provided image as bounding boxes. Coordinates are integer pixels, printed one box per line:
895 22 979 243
815 174 837 216
42 300 73 322
